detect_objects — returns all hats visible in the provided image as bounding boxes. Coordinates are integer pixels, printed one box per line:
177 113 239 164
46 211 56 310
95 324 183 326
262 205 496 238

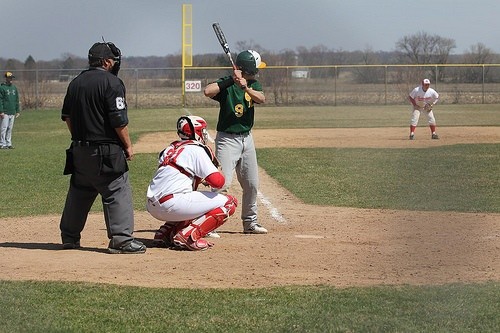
5 71 15 78
88 42 117 61
422 79 431 85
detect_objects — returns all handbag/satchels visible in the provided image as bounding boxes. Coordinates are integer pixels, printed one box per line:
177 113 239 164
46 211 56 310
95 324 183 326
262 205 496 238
95 144 130 185
63 141 74 175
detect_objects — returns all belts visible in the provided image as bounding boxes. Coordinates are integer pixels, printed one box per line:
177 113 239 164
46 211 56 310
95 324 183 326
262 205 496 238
76 141 99 149
153 194 174 206
226 131 250 136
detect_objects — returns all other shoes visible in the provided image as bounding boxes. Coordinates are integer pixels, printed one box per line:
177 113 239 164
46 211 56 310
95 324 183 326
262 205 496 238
0 145 4 149
432 134 438 139
410 135 414 140
5 146 15 149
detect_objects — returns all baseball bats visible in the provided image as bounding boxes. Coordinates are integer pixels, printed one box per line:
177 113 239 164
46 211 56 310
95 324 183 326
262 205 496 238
212 22 245 89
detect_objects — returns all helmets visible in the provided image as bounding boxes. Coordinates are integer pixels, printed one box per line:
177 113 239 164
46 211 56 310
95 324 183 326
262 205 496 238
236 50 267 75
177 115 209 144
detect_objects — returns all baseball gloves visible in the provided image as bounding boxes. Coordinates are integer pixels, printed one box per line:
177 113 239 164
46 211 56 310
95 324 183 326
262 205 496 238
424 103 432 112
202 156 221 187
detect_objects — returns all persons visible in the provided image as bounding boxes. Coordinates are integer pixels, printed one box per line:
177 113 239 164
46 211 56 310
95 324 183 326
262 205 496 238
407 78 440 140
0 71 20 148
59 40 149 253
147 115 239 251
204 49 269 237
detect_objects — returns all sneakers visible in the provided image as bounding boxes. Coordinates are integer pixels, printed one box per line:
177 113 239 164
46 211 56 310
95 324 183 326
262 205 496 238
108 240 146 253
243 223 268 234
204 230 220 239
173 232 209 251
154 230 175 247
61 235 81 249
